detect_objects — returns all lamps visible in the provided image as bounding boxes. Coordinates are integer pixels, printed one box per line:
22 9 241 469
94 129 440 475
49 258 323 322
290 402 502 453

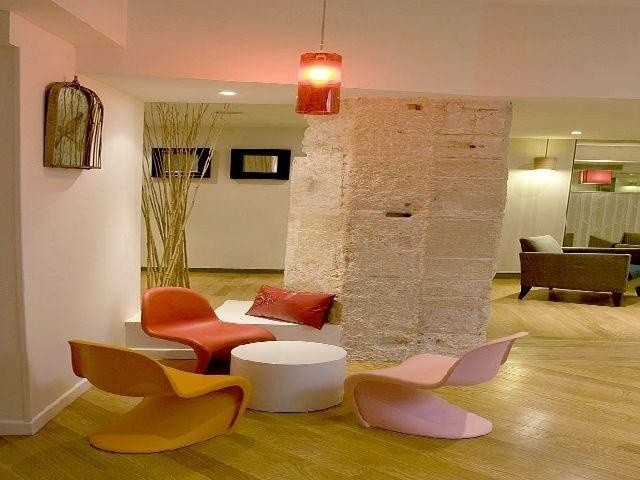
579 169 612 185
296 0 343 116
533 139 557 170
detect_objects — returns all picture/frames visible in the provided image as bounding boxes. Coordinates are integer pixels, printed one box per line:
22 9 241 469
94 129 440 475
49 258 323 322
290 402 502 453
230 149 291 180
152 148 211 178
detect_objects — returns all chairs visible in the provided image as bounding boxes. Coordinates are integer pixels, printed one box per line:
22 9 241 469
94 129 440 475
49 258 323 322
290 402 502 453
344 332 526 438
69 340 253 454
519 248 640 307
615 243 640 248
141 287 277 375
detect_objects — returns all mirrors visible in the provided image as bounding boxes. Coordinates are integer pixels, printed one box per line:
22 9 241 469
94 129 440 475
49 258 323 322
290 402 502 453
563 139 640 248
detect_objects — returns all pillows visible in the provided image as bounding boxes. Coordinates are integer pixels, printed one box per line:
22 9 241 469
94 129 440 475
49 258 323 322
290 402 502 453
245 284 335 330
624 232 640 245
519 234 563 254
